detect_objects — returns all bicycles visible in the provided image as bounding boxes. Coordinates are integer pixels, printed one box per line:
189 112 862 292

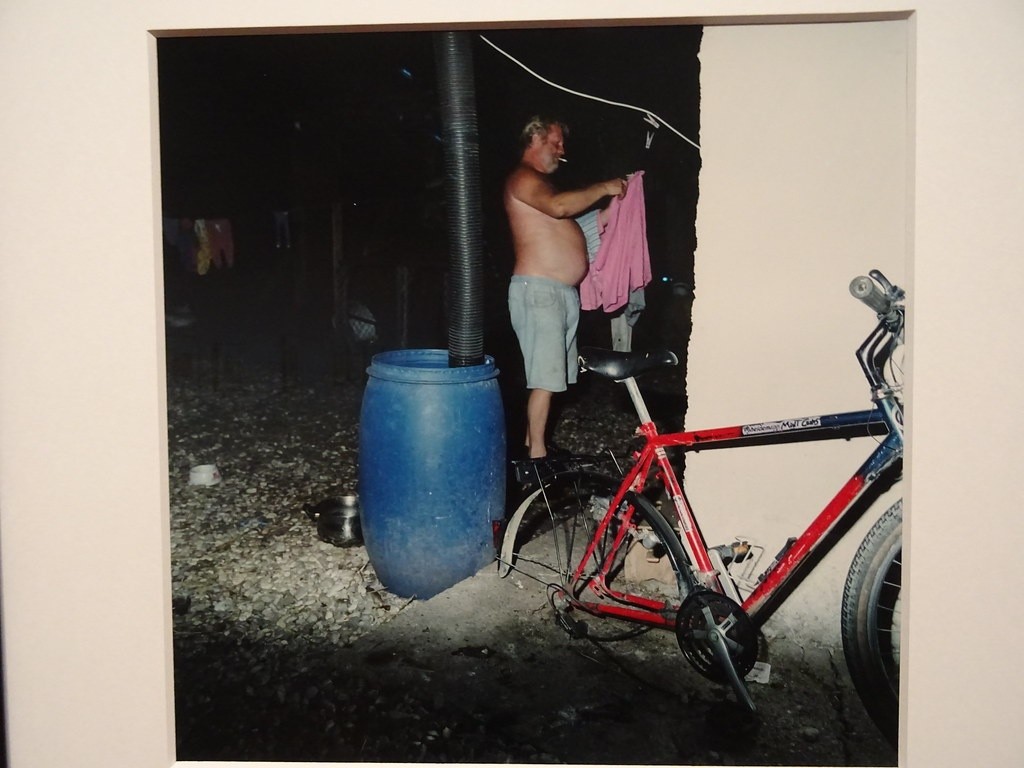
494 270 904 756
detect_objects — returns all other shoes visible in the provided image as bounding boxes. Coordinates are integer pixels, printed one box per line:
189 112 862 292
519 438 572 458
521 455 579 480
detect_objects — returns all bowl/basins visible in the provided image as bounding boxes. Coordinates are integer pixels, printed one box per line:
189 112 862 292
189 465 221 485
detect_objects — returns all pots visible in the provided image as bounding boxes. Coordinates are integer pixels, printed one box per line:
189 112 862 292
302 495 363 547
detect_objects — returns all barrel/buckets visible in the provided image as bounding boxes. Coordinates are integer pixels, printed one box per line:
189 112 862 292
357 348 507 600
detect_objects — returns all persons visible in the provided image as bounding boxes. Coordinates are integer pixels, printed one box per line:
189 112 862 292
504 116 628 484
